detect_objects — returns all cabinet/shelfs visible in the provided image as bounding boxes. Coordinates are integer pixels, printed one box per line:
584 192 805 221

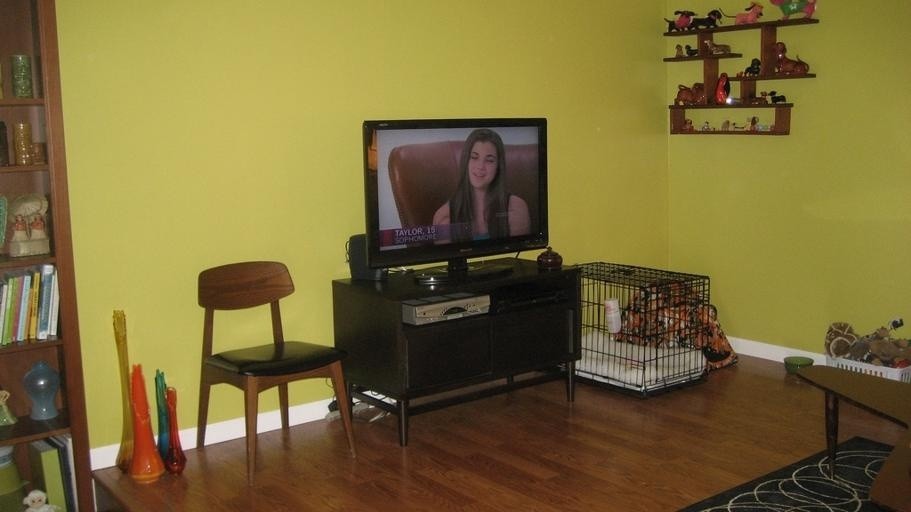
663 18 818 136
1 1 93 512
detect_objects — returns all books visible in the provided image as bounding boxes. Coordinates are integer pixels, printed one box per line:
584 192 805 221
0 264 60 345
23 433 79 512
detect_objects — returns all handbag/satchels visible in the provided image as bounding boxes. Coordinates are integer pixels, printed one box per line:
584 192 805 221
613 281 738 369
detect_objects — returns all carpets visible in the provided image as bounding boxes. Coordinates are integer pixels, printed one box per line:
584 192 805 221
676 438 895 512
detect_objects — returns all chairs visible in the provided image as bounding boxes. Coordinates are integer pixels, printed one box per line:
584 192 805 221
197 258 358 485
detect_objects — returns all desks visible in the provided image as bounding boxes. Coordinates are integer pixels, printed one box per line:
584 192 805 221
332 256 583 448
797 365 910 512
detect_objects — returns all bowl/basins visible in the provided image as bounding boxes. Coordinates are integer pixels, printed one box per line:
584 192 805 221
784 356 813 374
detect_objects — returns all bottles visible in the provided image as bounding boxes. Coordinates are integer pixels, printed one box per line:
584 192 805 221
604 296 622 334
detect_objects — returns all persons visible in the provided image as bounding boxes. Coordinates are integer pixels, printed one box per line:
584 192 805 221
12 214 46 241
433 129 532 244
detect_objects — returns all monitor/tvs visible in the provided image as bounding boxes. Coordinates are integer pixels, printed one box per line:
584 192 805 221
361 115 550 286
